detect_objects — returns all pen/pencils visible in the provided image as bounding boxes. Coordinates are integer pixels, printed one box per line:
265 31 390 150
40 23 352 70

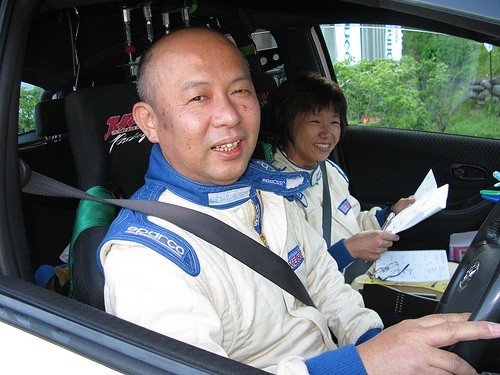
363 212 396 263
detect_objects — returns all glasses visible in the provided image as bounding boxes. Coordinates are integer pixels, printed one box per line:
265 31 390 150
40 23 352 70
374 262 410 281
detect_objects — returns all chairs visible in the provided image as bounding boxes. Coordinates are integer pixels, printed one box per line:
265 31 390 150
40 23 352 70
62 79 152 312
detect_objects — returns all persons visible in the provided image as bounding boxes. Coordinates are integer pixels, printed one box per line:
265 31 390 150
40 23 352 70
259 73 440 330
95 27 500 375
